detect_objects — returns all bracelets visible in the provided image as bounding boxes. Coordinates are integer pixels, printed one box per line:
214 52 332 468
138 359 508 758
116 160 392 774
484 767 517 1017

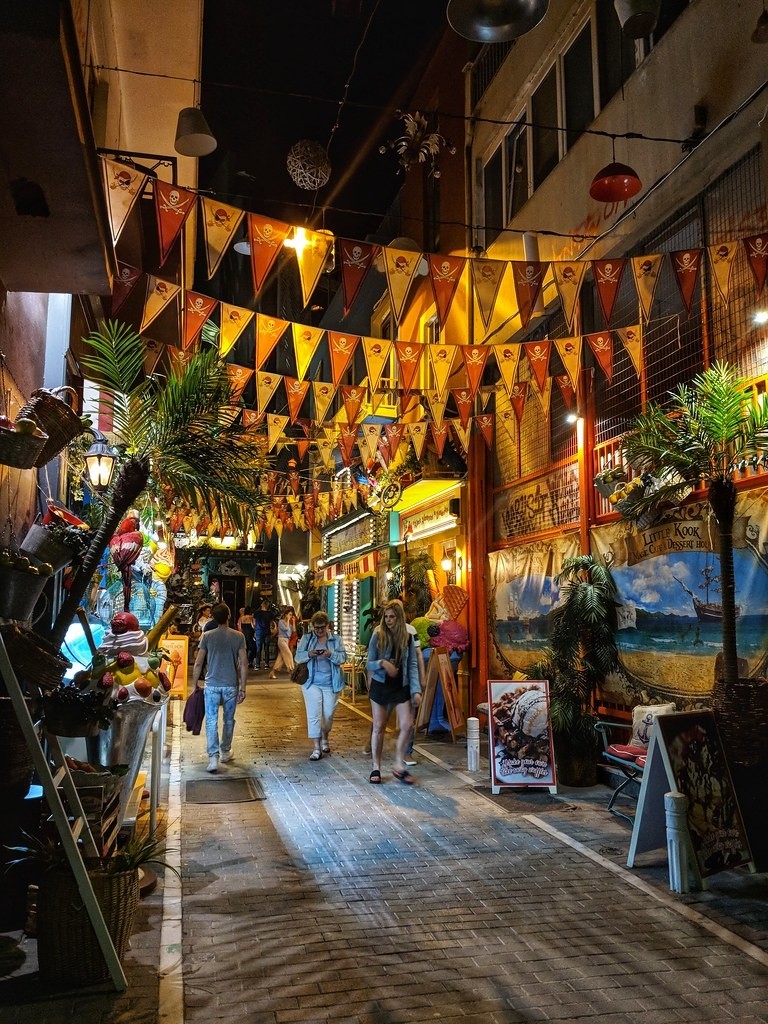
240 689 246 693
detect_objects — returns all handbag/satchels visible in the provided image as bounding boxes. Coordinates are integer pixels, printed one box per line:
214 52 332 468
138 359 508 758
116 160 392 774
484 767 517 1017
291 663 308 685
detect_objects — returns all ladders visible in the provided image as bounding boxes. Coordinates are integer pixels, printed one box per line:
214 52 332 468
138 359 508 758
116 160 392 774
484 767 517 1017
0 616 132 995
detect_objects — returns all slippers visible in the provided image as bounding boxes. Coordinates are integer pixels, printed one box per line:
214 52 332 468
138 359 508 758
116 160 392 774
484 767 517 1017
369 770 382 784
393 770 414 784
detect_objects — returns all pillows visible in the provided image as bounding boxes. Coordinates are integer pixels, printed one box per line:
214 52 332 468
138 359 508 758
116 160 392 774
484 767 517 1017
628 701 674 751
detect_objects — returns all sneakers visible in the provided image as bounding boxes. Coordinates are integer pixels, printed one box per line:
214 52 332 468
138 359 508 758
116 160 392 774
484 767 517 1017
207 760 218 771
220 749 234 762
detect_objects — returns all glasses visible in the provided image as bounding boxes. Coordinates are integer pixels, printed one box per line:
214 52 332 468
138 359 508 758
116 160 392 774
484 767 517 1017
313 626 326 630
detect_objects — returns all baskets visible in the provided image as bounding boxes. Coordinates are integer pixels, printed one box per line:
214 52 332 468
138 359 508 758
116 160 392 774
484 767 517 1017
0 713 39 802
7 623 71 690
38 859 138 984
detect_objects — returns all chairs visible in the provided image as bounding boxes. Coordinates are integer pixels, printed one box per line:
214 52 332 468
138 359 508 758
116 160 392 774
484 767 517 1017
340 640 370 705
594 701 681 822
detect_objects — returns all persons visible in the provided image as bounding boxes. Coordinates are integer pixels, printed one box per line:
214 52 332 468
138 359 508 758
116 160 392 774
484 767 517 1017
295 610 347 761
236 606 256 669
198 603 213 680
365 600 426 786
204 602 222 632
251 601 277 670
192 603 248 772
267 606 299 679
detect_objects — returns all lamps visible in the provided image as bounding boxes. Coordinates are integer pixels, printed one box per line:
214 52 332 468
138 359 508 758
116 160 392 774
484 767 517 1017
565 409 582 422
445 1 550 44
69 420 116 492
174 81 222 155
439 545 452 570
385 562 393 579
588 136 642 204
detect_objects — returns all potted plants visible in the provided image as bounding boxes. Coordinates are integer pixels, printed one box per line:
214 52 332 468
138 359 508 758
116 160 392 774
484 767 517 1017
526 552 628 788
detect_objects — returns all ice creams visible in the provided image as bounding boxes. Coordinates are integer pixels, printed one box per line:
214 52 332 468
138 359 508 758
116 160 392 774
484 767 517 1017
74 612 171 830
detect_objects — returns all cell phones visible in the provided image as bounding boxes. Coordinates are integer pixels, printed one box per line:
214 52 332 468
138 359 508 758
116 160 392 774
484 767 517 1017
316 650 325 656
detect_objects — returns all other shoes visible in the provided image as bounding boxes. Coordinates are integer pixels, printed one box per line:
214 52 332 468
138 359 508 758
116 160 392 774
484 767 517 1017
309 747 322 760
402 755 418 766
363 738 372 755
270 672 278 679
322 742 330 753
265 664 270 669
254 666 260 670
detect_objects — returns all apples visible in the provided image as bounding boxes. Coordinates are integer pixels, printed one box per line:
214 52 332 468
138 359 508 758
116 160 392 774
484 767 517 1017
0 414 43 437
0 550 53 577
